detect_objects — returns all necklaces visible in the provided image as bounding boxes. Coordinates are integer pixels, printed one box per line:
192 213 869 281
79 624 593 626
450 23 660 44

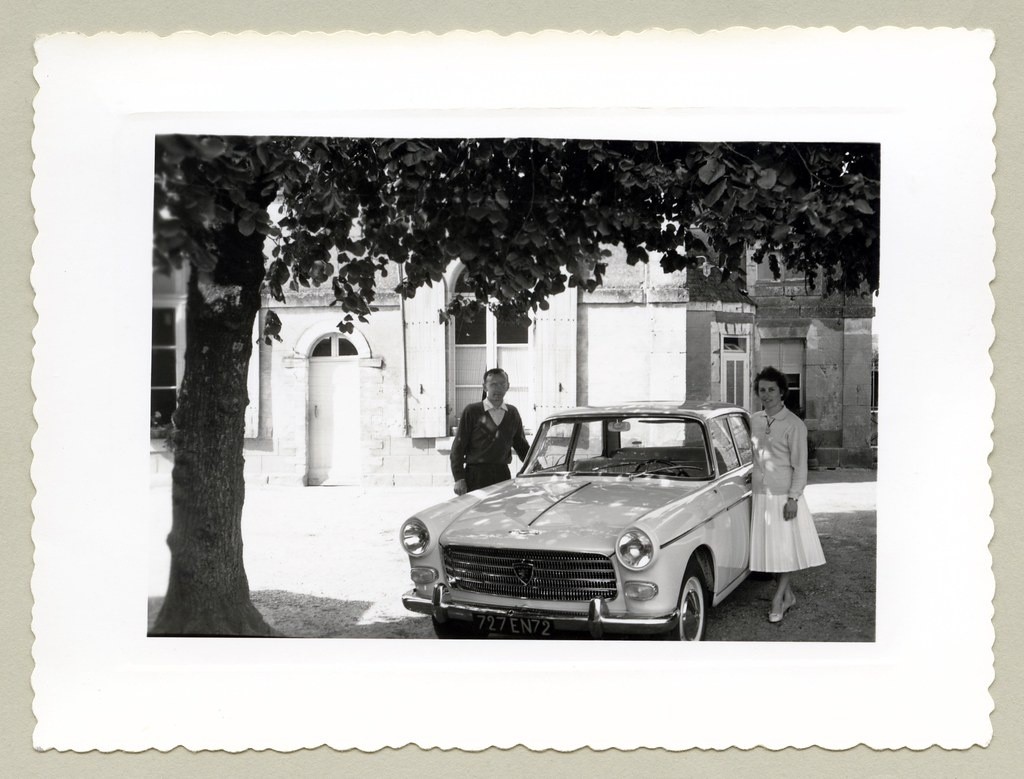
765 416 775 434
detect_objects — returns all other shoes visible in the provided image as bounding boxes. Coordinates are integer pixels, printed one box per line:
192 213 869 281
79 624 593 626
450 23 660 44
768 594 796 614
769 600 784 622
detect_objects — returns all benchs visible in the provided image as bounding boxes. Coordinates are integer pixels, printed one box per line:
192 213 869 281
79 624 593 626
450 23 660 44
572 458 721 477
612 447 724 460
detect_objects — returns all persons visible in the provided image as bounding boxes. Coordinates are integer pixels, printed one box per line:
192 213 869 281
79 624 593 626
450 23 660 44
450 369 544 495
748 366 828 622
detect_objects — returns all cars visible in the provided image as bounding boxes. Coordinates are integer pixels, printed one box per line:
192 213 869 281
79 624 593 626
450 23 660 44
398 401 754 640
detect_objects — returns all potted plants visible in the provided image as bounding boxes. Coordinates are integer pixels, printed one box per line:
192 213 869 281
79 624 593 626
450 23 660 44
807 436 825 472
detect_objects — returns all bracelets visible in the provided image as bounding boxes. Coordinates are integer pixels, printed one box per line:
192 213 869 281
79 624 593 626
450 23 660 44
788 497 798 501
